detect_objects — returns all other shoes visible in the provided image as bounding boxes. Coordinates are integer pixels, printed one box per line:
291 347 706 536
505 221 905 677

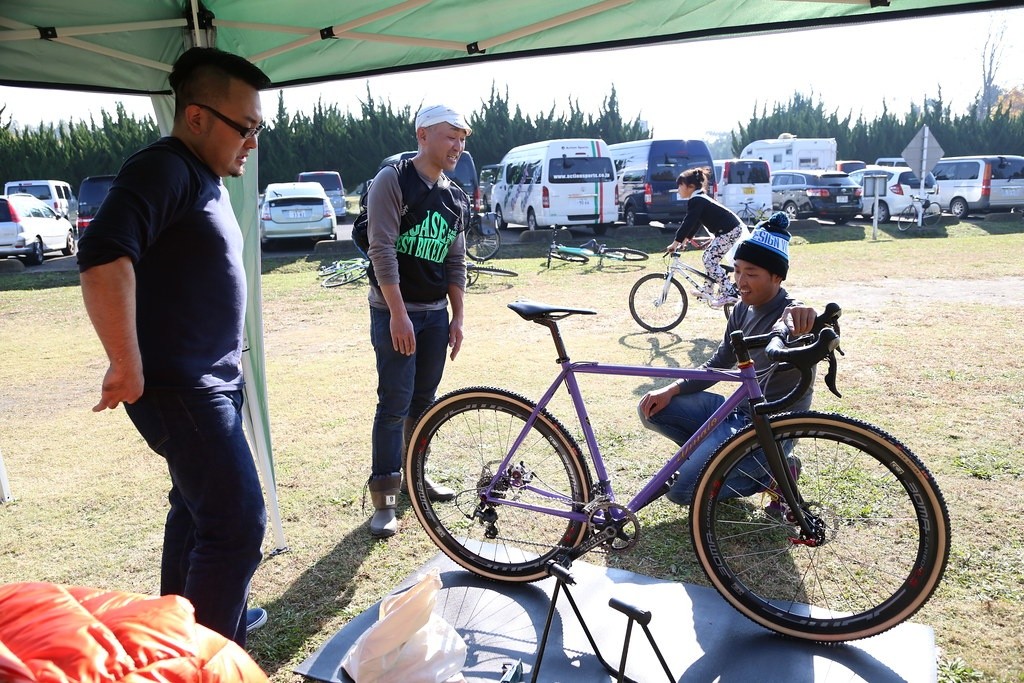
711 294 738 306
691 288 714 299
245 607 267 636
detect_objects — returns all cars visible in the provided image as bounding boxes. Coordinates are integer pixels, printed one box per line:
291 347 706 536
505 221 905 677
0 133 1024 269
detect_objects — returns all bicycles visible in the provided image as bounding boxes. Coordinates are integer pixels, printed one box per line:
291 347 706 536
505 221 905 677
400 302 951 645
318 204 650 290
627 242 742 334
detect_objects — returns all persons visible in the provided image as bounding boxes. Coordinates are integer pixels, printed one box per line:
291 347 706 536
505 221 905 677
352 105 472 537
668 168 744 306
638 213 817 516
75 46 272 650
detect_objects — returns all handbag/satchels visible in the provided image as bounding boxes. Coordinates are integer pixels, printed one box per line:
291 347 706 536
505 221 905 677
351 173 445 261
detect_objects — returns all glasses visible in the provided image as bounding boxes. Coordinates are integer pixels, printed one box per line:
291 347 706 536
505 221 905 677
191 103 266 139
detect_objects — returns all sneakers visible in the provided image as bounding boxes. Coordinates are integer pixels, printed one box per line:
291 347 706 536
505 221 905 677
764 456 801 516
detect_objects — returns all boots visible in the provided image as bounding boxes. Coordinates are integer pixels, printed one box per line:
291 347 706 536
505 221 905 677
368 472 401 536
400 448 455 501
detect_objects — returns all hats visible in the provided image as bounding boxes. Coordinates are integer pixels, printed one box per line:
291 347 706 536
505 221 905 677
415 104 472 138
734 211 791 281
